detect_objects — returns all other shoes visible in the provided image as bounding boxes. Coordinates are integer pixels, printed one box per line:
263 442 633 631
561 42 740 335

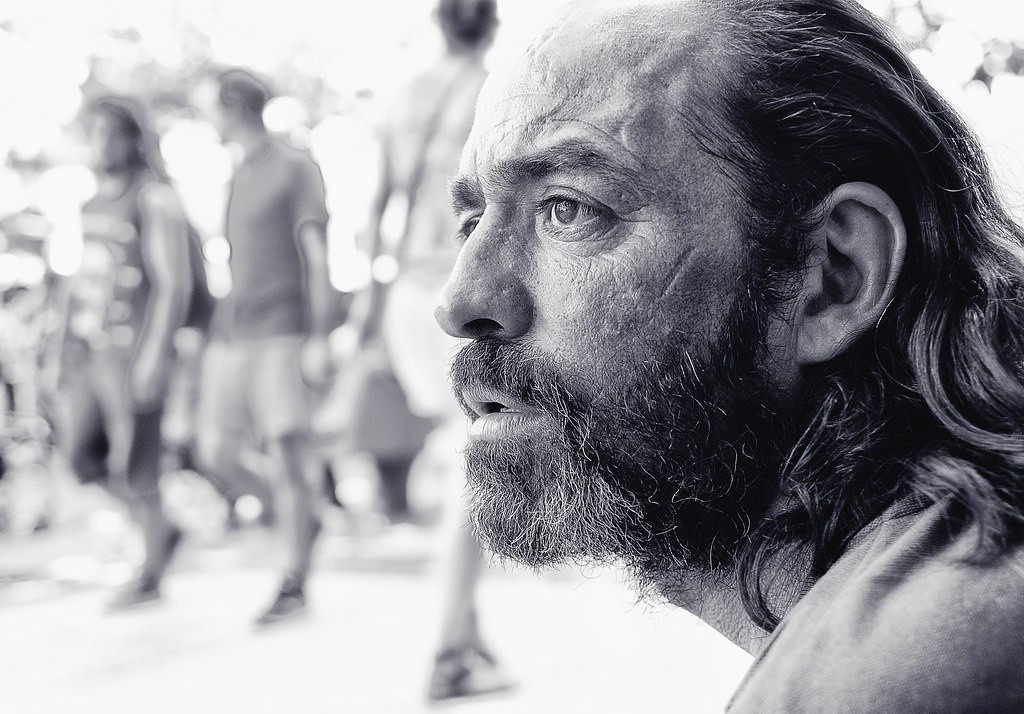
255 587 301 627
430 644 490 694
116 524 182 607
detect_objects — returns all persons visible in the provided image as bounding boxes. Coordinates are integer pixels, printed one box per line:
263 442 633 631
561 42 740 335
431 1 1024 714
53 96 218 612
334 0 516 701
201 66 335 627
